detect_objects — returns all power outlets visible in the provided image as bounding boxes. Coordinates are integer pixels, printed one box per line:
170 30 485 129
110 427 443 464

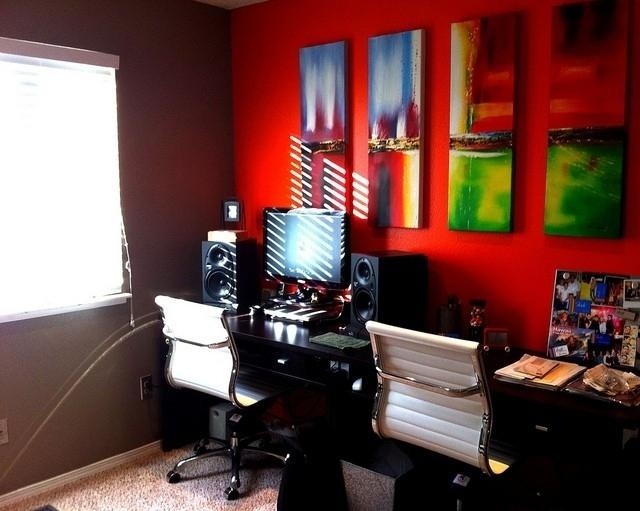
1 418 8 447
139 372 155 405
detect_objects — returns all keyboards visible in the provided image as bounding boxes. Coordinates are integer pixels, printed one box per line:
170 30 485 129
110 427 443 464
264 305 327 323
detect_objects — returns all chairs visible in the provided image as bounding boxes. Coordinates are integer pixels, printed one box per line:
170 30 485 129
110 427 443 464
155 294 288 502
363 318 519 475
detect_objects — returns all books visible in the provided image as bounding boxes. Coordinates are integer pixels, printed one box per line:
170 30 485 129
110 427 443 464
493 352 640 410
307 332 371 353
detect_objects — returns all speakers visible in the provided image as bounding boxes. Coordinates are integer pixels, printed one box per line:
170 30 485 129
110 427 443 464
201 239 262 315
350 250 429 341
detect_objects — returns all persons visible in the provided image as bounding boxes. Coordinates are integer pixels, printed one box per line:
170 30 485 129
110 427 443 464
547 271 640 372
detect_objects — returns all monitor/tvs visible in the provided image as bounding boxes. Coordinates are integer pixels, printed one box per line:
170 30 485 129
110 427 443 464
262 208 348 303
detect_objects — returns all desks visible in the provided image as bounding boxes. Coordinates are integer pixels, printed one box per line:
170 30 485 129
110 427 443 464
154 276 426 462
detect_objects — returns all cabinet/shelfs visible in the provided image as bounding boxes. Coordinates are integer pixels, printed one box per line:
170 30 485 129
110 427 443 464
465 333 640 459
